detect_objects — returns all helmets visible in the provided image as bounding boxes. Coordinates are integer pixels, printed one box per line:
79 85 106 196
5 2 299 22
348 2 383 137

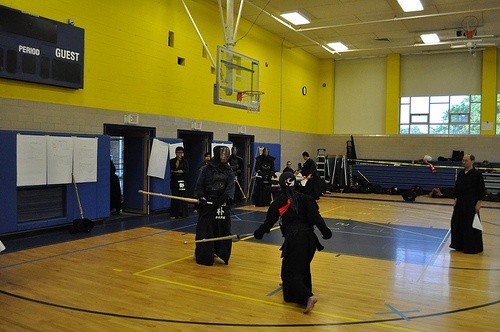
213 146 230 164
279 172 296 192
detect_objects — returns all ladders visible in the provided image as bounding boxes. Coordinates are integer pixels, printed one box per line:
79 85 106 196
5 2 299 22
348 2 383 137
314 148 327 179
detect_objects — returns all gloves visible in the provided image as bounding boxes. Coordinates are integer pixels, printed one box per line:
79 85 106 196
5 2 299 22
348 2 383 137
214 198 223 208
200 197 207 206
322 231 332 239
253 228 265 239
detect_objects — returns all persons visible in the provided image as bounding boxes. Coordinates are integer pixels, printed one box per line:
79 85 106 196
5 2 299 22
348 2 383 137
254 172 332 314
169 147 190 219
229 147 243 204
297 152 331 200
194 146 236 265
448 152 488 255
254 148 276 207
193 152 211 214
109 157 122 213
283 161 296 175
